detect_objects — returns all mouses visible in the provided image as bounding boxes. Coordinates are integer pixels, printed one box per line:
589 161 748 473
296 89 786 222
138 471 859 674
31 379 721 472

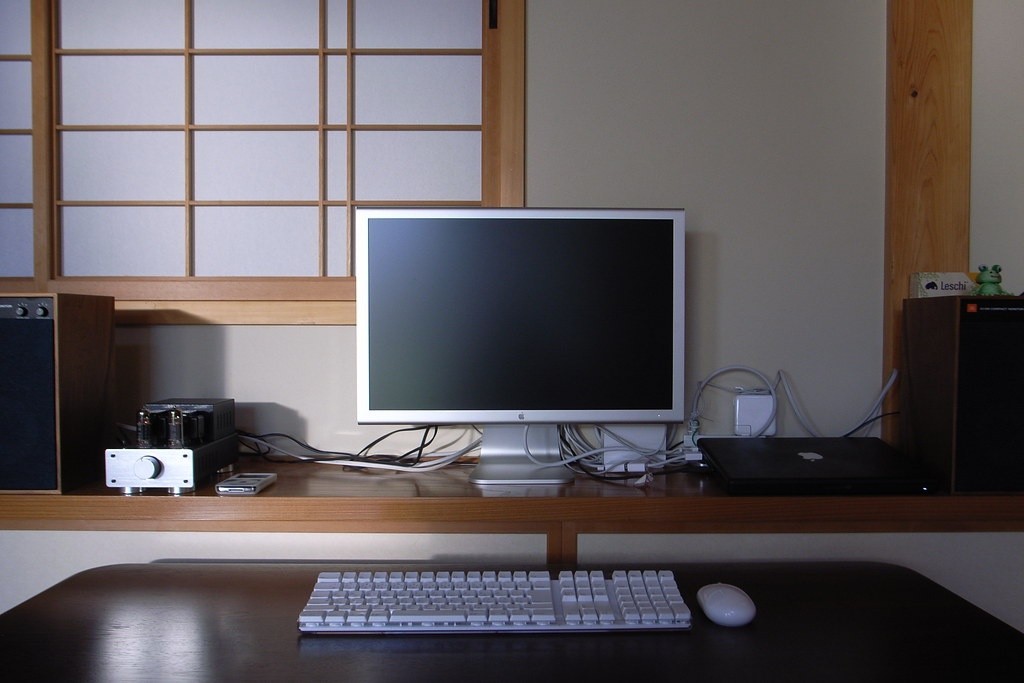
697 582 756 628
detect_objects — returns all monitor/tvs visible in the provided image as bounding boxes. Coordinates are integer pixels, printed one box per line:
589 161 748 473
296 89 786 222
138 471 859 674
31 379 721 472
355 205 687 484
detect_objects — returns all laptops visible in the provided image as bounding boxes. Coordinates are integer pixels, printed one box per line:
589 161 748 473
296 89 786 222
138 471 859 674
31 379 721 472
688 437 939 494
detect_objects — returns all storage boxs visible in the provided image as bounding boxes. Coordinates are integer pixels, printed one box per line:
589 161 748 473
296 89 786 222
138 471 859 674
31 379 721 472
909 272 979 298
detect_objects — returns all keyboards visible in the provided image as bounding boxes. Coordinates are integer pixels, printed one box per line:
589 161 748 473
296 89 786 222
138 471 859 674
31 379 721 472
296 570 693 635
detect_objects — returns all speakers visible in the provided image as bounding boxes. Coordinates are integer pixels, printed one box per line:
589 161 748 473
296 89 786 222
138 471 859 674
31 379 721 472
0 292 115 496
902 296 1024 496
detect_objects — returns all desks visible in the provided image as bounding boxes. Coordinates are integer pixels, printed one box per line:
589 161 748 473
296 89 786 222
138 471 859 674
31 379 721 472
0 456 1024 632
0 564 1024 683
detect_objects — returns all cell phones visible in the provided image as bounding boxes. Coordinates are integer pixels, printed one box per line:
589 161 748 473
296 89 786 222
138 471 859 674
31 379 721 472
215 472 278 494
